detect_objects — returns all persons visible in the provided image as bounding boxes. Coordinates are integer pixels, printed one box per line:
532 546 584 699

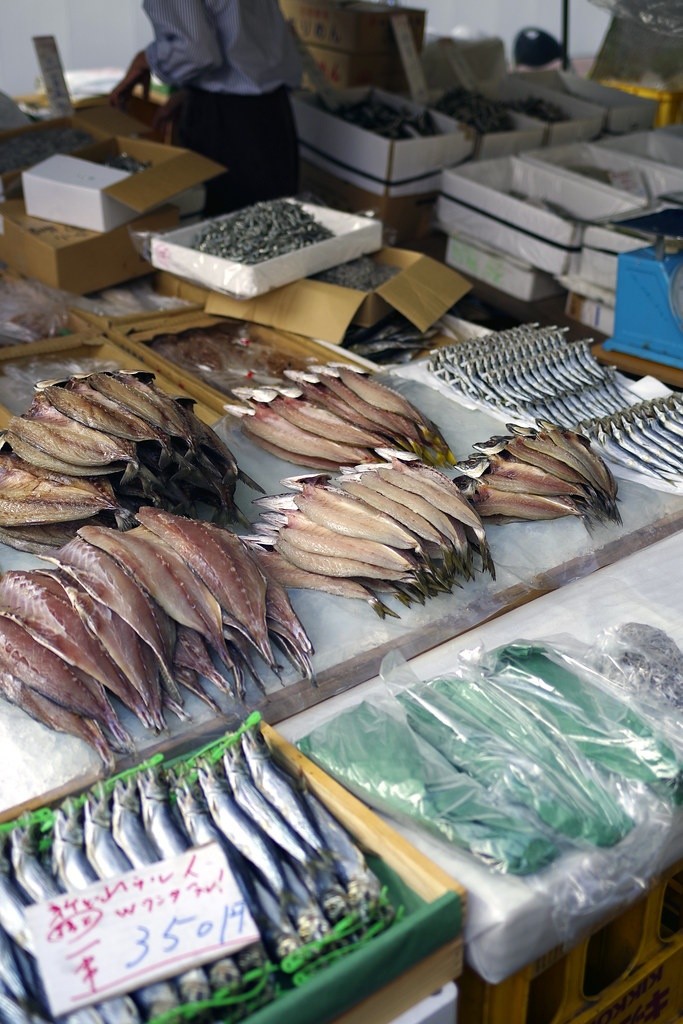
107 0 305 218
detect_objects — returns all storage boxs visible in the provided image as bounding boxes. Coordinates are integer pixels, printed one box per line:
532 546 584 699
0 0 682 1024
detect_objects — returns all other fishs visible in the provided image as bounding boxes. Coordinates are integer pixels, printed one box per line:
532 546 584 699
0 79 683 1024
